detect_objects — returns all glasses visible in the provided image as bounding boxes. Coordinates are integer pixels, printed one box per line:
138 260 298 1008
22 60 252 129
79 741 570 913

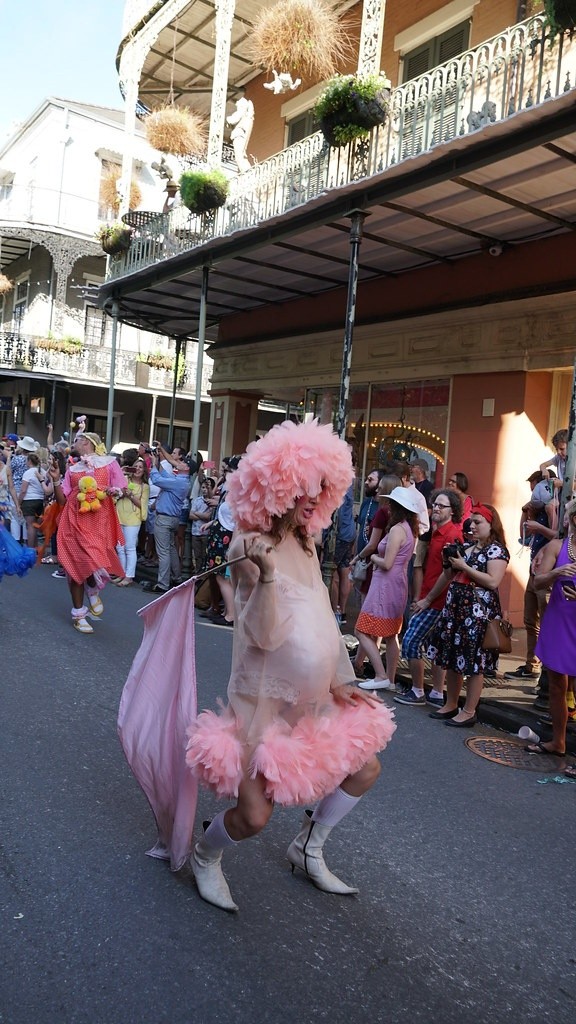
74 437 84 443
365 477 378 482
448 478 458 484
408 465 420 471
432 502 451 510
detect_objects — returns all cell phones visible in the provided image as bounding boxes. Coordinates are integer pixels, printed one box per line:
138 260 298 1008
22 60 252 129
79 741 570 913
560 580 576 595
152 442 158 447
125 467 136 473
10 445 16 456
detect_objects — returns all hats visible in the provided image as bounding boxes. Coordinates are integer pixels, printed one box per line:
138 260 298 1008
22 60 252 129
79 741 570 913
17 436 37 451
8 435 19 442
378 487 419 514
181 456 197 475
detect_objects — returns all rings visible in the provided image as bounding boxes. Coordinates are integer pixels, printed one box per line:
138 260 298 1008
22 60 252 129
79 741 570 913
256 544 260 547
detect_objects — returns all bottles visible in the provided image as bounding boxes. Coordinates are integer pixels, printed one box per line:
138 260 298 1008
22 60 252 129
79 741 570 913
334 605 342 631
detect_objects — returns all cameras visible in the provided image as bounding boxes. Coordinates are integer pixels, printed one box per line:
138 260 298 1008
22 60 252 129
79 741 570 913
443 542 465 560
145 448 156 456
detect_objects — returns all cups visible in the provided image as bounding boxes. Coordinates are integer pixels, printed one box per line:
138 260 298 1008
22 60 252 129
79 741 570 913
519 726 540 744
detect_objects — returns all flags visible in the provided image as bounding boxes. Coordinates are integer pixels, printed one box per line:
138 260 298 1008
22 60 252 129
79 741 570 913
117 577 197 873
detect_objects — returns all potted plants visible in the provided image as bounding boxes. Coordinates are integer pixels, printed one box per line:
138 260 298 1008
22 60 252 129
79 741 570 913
179 168 229 214
526 0 576 53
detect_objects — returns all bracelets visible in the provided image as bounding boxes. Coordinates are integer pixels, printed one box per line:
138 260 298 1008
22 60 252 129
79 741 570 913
259 576 274 583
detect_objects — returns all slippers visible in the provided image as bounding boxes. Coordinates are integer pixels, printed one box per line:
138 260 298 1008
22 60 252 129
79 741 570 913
566 766 576 779
525 742 567 757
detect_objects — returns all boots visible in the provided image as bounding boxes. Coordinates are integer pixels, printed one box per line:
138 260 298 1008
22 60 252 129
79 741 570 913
189 820 242 911
285 809 360 894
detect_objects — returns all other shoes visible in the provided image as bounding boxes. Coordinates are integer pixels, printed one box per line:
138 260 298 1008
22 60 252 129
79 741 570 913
484 670 496 677
335 612 347 626
2 541 236 633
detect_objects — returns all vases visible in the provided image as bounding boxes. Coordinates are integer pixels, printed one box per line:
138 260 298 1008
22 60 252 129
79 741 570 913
322 93 386 147
101 231 133 255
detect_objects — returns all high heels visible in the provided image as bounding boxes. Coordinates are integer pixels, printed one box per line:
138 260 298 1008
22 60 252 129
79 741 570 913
429 707 459 719
444 710 476 728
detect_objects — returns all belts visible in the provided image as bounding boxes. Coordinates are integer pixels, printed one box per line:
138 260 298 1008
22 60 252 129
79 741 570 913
157 512 178 518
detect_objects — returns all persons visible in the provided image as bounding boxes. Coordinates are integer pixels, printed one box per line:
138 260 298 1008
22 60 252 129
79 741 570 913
228 91 254 171
328 429 576 778
152 151 181 186
0 416 237 629
47 430 133 633
189 427 382 910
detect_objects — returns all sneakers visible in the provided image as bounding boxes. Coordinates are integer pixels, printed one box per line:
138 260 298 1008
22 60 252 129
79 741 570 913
539 705 576 724
533 692 551 711
504 670 541 680
532 680 542 694
349 659 444 708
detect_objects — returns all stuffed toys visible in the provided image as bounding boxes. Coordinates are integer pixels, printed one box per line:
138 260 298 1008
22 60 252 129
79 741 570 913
77 476 106 512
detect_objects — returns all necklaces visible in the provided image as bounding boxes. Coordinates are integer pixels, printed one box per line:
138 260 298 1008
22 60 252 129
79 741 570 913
567 533 576 561
362 498 373 544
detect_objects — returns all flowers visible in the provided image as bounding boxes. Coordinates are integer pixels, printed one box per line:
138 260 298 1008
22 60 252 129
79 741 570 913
94 219 126 245
308 71 391 152
135 352 187 388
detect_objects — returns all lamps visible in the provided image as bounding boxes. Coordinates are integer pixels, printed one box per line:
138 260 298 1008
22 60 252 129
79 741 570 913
375 383 419 468
13 393 25 424
135 409 145 440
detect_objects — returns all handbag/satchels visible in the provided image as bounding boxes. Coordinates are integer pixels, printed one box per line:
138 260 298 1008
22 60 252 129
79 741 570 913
353 559 367 580
481 619 512 653
